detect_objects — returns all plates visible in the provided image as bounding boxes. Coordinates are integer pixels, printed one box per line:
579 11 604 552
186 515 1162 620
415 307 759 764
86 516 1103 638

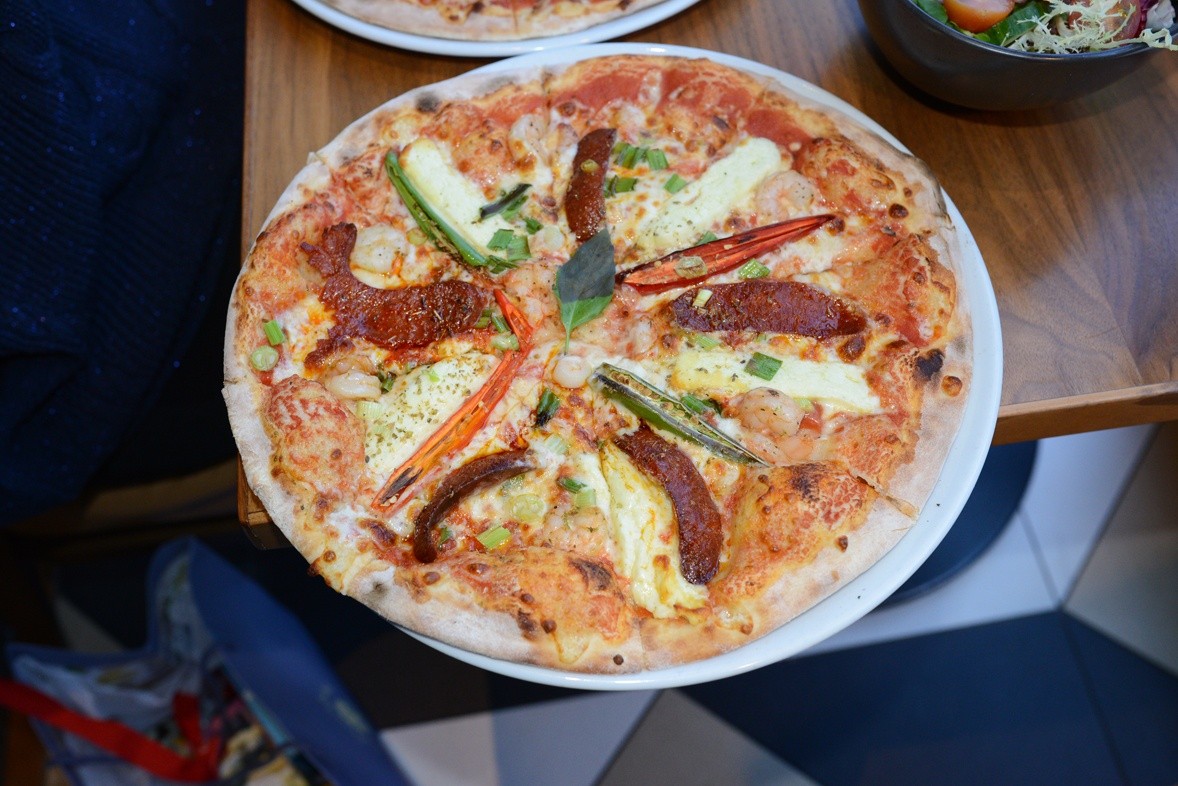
295 1 701 58
256 44 1004 692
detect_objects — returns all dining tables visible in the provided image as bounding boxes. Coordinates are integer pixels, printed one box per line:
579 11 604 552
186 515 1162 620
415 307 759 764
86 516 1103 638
235 2 1174 546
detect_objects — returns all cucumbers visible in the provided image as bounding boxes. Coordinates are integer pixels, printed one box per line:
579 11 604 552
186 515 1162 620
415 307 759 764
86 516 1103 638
986 2 1050 48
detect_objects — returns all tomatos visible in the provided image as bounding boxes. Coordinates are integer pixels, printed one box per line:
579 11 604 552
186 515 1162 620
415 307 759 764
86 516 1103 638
1067 0 1144 42
944 0 1018 33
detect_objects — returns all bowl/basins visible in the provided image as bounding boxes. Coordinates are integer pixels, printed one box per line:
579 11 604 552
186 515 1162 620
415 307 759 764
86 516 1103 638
855 0 1177 110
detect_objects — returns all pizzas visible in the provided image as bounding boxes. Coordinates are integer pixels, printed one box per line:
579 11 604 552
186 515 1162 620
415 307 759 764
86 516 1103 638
223 42 972 679
323 1 664 40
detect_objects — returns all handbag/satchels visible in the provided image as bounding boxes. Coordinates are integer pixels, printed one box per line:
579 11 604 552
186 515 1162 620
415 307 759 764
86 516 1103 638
0 537 413 786
